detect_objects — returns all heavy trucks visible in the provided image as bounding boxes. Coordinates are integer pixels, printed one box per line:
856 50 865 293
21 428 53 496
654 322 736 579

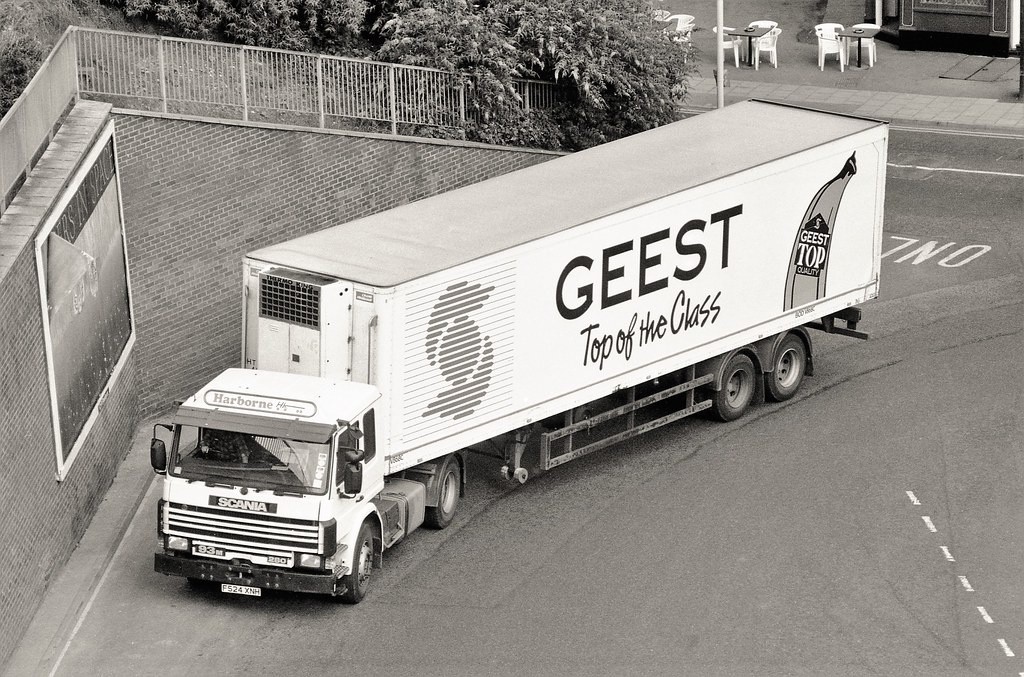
156 97 891 607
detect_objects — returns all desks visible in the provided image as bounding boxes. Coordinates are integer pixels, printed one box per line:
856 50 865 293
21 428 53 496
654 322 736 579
727 27 773 68
654 21 673 31
838 28 881 70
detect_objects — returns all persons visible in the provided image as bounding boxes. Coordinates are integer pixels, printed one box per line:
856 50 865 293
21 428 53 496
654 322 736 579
199 429 249 463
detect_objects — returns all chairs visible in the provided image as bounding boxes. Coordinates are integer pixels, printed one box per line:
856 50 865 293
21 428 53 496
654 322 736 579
815 23 848 67
744 21 779 65
654 10 697 63
817 30 845 72
713 26 746 68
846 24 880 67
750 28 782 71
